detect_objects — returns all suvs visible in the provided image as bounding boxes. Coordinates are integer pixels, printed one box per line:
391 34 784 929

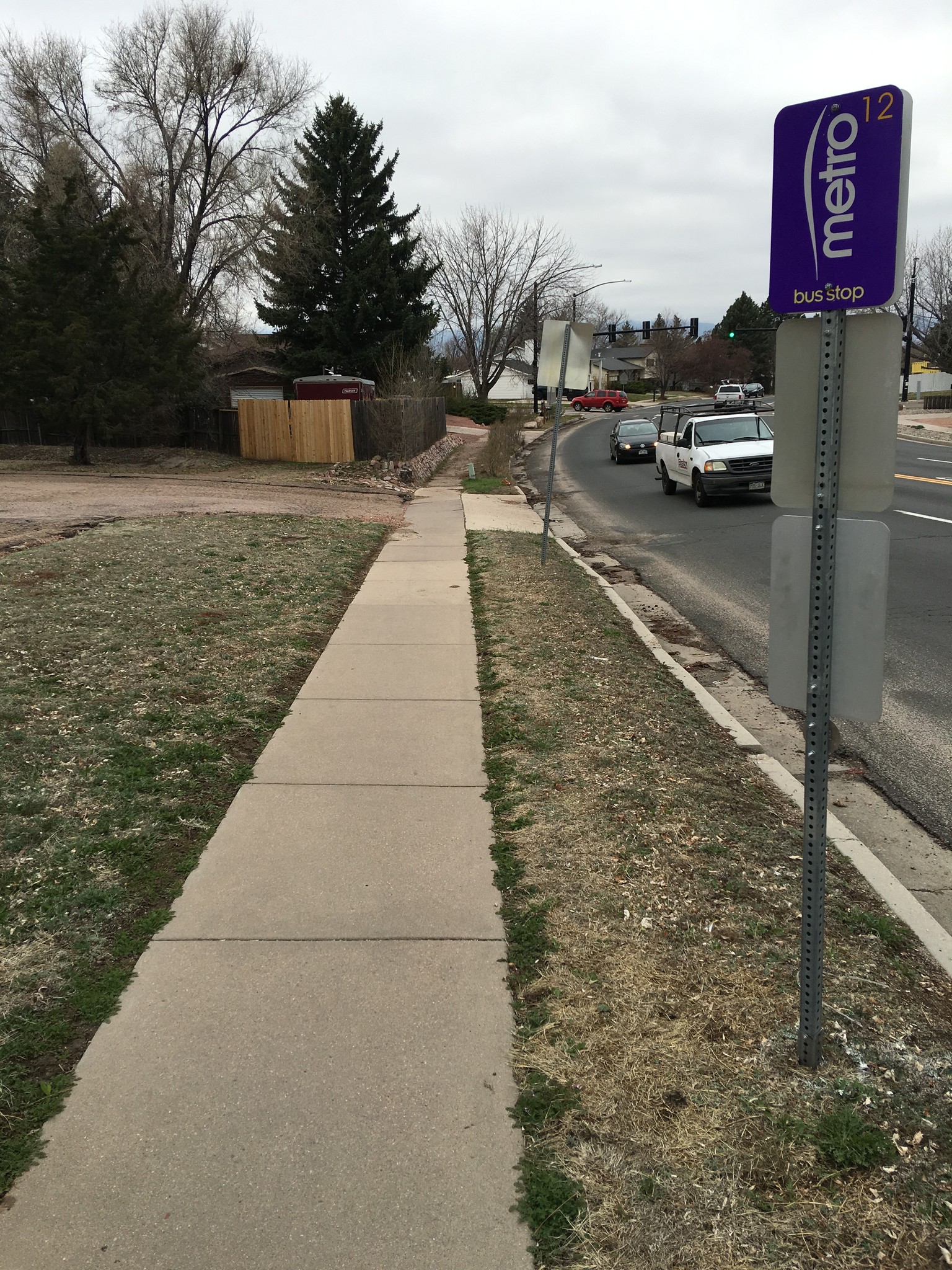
570 390 629 413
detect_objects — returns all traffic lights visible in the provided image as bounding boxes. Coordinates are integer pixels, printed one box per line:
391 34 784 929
728 322 736 338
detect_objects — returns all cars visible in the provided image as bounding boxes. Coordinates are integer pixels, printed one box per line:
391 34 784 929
742 383 765 398
609 416 666 465
532 385 596 402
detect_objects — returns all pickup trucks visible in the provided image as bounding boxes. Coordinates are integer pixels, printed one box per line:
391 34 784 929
653 397 774 509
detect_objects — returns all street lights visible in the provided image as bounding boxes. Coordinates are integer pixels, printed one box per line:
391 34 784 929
532 264 602 413
573 279 632 321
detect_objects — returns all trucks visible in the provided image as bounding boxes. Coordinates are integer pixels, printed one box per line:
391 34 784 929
712 384 748 411
